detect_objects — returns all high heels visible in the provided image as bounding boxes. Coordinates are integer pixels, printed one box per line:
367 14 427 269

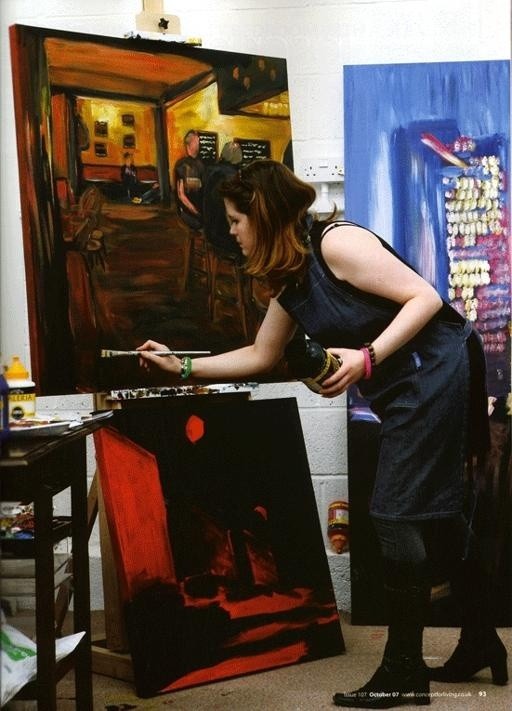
334 654 433 708
429 632 509 685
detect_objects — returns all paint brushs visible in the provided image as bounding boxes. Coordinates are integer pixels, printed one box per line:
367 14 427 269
101 350 211 359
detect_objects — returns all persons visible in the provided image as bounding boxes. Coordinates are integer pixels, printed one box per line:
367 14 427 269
170 130 212 237
202 141 244 248
132 160 510 710
119 152 148 203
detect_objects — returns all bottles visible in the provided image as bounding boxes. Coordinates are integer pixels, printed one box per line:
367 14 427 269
3 356 37 424
284 337 342 395
327 500 350 555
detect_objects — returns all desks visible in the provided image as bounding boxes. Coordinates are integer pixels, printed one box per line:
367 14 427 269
0 411 116 711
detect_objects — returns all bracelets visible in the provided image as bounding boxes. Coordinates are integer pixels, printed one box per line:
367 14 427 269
362 342 377 368
359 348 373 382
178 356 191 382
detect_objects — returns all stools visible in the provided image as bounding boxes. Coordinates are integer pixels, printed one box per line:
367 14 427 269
85 230 109 271
175 209 250 341
